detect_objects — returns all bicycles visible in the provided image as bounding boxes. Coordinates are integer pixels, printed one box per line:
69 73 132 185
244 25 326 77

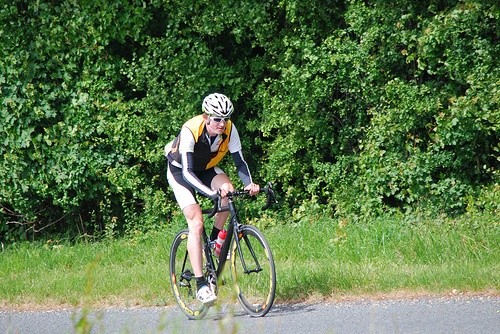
169 182 279 320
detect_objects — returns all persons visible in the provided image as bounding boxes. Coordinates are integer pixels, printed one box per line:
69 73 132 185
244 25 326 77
164 93 260 303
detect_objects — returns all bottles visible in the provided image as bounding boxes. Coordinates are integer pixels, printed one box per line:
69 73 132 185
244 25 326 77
214 228 227 256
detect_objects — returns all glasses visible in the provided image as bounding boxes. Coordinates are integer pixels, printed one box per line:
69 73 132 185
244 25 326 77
207 117 230 123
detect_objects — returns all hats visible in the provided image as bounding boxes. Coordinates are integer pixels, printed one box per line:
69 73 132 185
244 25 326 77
202 93 234 118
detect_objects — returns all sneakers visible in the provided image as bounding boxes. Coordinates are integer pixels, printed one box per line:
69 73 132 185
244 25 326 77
208 236 231 260
195 286 216 303
